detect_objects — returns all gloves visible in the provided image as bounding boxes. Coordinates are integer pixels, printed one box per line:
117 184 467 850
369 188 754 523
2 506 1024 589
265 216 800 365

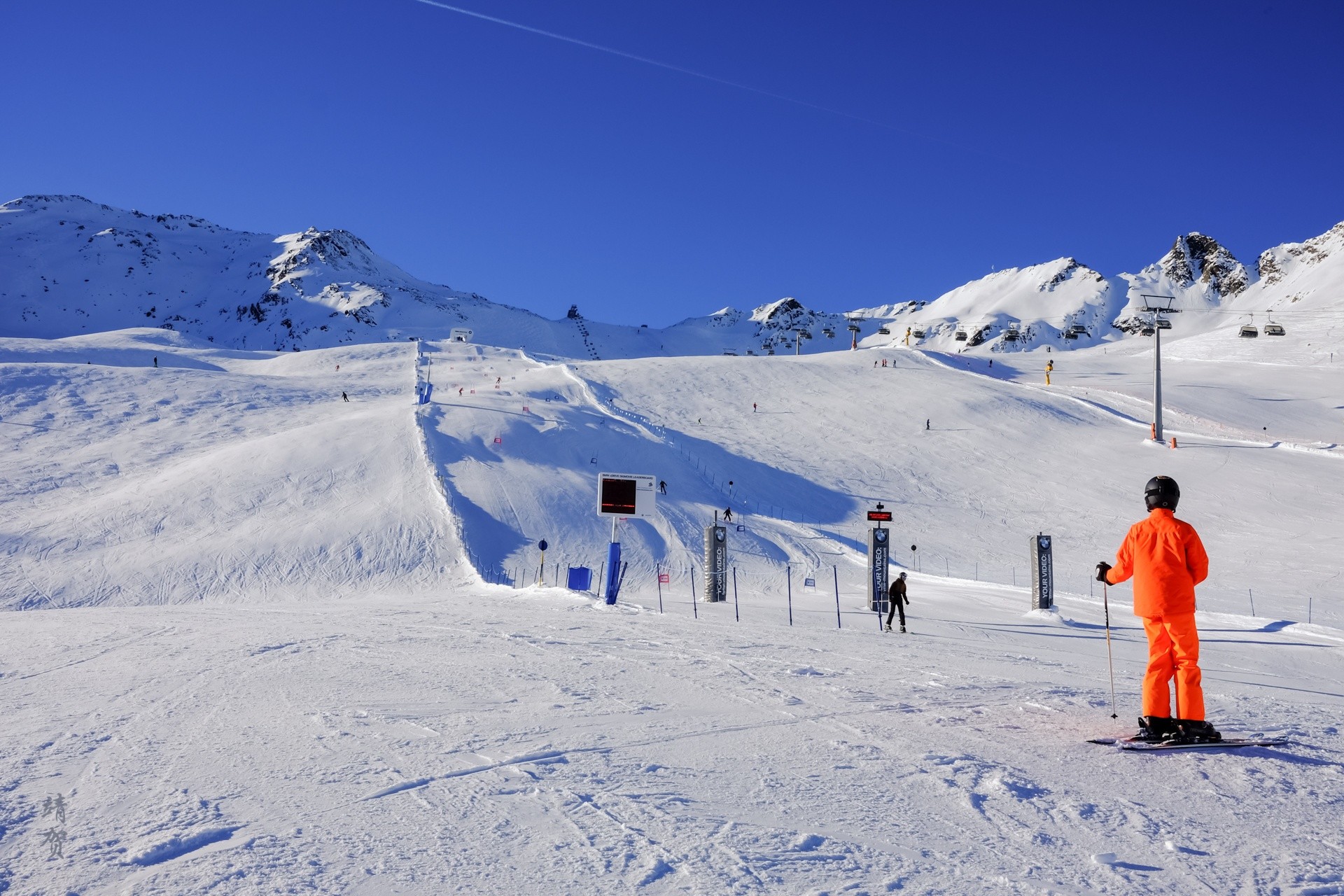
906 601 909 605
1095 561 1114 586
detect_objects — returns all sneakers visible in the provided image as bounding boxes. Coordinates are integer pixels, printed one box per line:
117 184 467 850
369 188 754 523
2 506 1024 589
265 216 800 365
1178 722 1221 743
1144 716 1179 738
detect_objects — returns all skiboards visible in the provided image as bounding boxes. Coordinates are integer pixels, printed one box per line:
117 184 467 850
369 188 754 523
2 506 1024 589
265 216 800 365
885 629 914 635
1085 733 1290 751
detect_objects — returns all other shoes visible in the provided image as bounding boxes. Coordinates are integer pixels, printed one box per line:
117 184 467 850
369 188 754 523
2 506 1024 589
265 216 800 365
900 626 905 630
884 625 891 630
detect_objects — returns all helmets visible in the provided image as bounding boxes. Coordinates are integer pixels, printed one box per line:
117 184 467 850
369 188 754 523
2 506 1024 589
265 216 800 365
1145 475 1180 513
899 572 907 581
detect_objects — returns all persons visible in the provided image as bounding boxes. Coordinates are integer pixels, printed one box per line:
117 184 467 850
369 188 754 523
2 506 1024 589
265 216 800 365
874 361 877 367
335 365 340 371
496 377 501 384
926 419 930 429
659 480 667 496
342 392 349 402
1094 475 1221 741
893 359 896 367
989 359 992 367
754 402 757 412
698 418 701 424
883 572 909 632
459 387 464 396
882 358 887 367
723 507 732 522
